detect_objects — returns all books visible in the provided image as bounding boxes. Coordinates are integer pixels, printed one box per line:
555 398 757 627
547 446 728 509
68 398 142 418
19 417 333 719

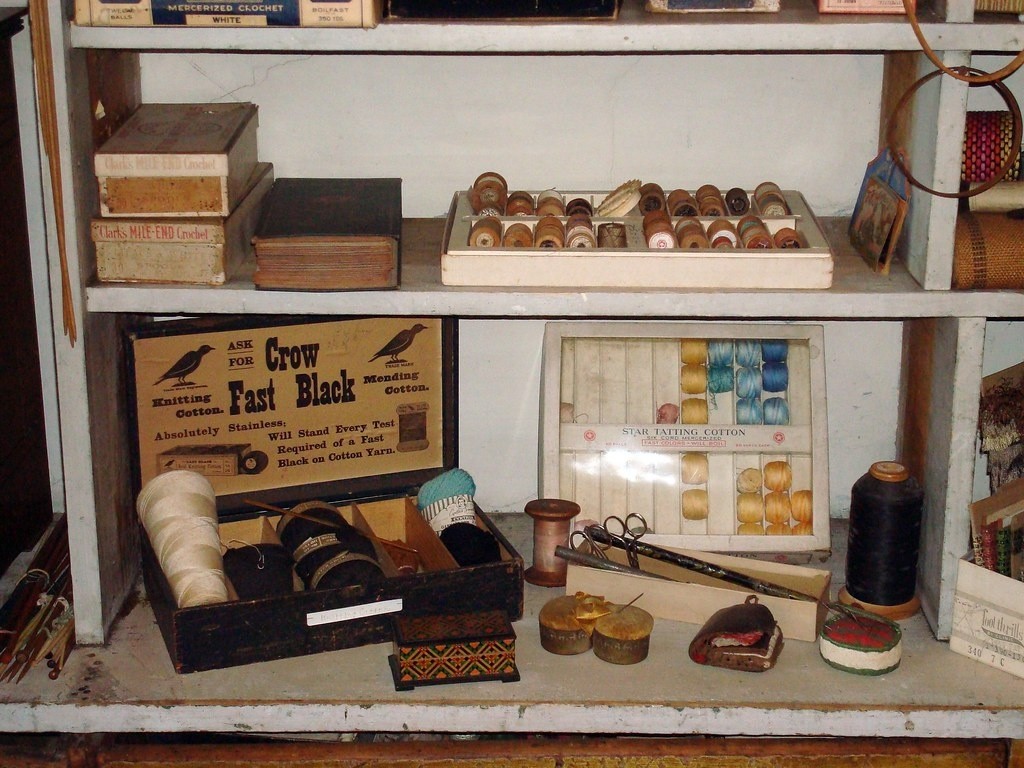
252 178 403 291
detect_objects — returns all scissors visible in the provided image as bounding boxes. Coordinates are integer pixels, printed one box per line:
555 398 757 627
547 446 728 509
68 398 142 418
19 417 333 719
603 513 648 569
570 524 612 561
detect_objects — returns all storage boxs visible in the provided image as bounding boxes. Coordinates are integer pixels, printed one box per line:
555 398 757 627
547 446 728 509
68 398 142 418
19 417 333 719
441 190 834 289
538 322 830 551
94 100 261 216
949 548 1024 678
124 314 523 673
388 608 519 691
91 162 274 285
566 538 832 641
73 0 382 28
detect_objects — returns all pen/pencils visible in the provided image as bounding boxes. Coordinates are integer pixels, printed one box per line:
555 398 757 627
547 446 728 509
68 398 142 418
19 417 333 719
584 525 818 602
554 544 682 583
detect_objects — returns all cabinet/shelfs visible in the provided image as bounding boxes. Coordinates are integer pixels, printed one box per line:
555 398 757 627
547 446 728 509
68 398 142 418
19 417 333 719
0 0 1024 768
